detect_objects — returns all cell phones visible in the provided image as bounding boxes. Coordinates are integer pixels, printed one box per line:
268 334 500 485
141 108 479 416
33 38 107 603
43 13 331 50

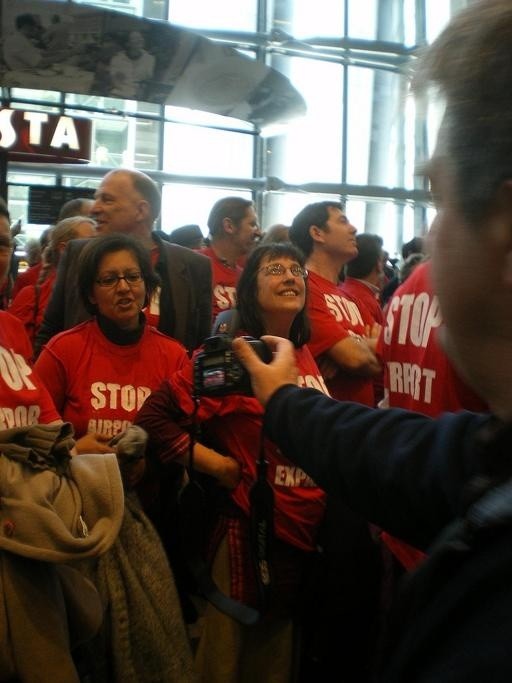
191 331 273 406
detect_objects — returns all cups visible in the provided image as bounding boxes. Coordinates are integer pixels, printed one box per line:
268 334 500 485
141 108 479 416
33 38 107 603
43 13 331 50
92 269 146 288
255 262 310 280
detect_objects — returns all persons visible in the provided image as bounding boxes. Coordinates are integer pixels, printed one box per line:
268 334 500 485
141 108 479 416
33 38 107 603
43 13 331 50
259 222 290 245
191 196 262 329
24 238 41 267
373 261 487 592
57 198 96 223
33 230 189 538
36 168 211 362
11 228 54 301
210 224 291 336
397 253 425 285
0 207 78 463
132 243 335 587
379 236 425 308
13 216 97 340
339 233 385 325
288 201 383 411
231 0 512 682
